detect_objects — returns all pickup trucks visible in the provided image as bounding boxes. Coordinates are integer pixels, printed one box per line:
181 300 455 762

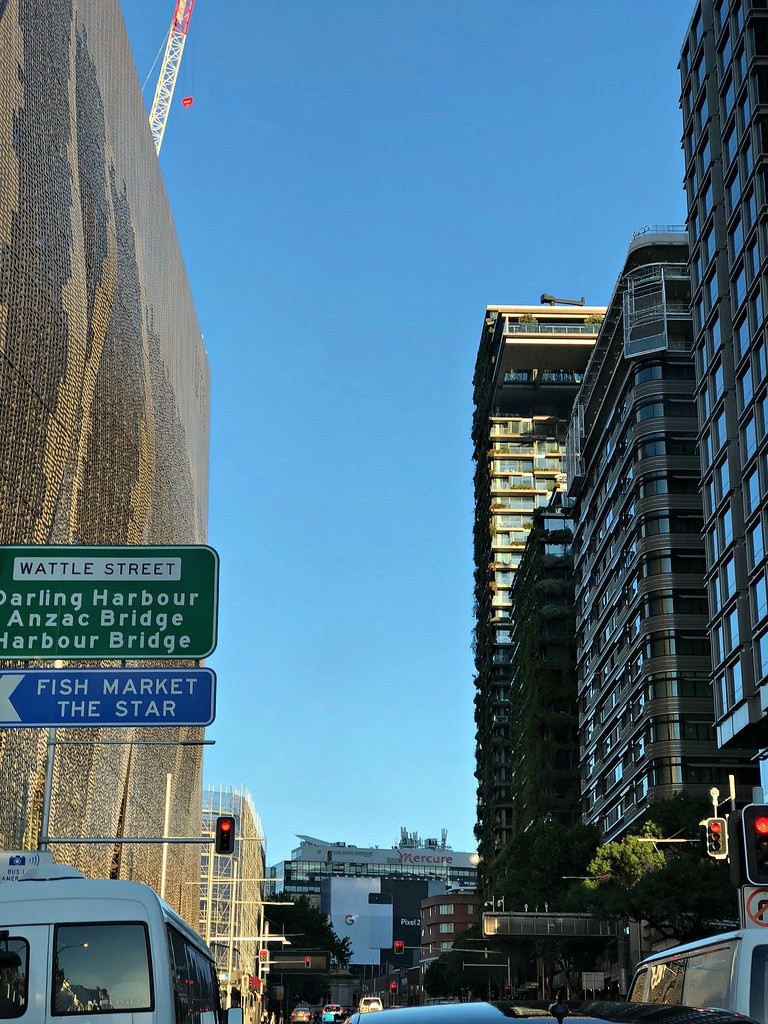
321 1004 343 1022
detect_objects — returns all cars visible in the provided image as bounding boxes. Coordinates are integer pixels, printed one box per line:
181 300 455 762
290 1008 314 1024
344 1008 357 1016
345 990 763 1024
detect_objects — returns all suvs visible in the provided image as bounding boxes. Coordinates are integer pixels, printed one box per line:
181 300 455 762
357 997 383 1013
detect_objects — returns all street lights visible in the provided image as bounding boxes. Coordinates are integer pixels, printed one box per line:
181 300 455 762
57 943 88 954
37 740 217 852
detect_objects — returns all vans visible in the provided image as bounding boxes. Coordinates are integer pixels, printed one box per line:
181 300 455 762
0 864 244 1024
625 928 768 1024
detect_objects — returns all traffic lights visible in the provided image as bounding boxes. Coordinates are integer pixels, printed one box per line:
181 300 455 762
215 816 236 855
504 985 511 995
242 975 249 988
742 804 768 886
394 941 404 955
305 957 311 968
390 983 398 994
705 818 729 859
259 949 268 963
